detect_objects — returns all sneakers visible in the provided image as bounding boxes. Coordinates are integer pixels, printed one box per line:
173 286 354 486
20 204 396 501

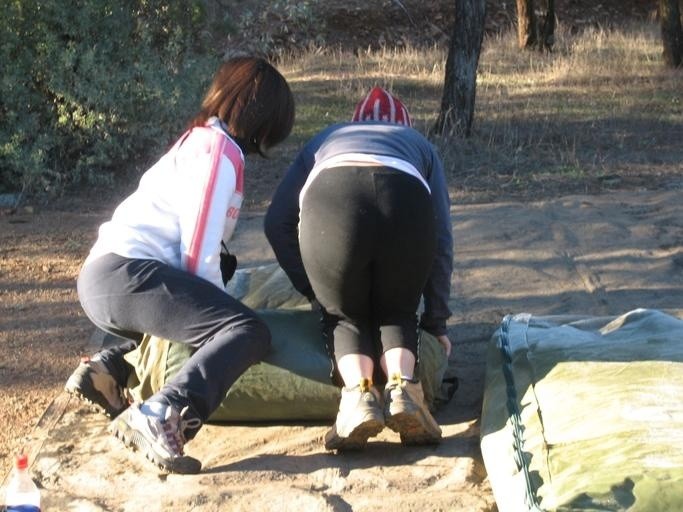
324 376 384 451
64 356 131 420
384 378 443 446
107 399 202 473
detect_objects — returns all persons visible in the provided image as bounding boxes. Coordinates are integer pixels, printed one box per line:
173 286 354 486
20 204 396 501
64 56 296 474
261 85 453 453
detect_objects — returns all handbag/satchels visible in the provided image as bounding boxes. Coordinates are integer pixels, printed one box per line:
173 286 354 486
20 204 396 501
219 252 238 288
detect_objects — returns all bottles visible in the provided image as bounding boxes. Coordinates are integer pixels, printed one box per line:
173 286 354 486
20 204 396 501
4 454 40 512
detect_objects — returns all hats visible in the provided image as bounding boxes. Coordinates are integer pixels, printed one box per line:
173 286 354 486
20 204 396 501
351 84 412 128
